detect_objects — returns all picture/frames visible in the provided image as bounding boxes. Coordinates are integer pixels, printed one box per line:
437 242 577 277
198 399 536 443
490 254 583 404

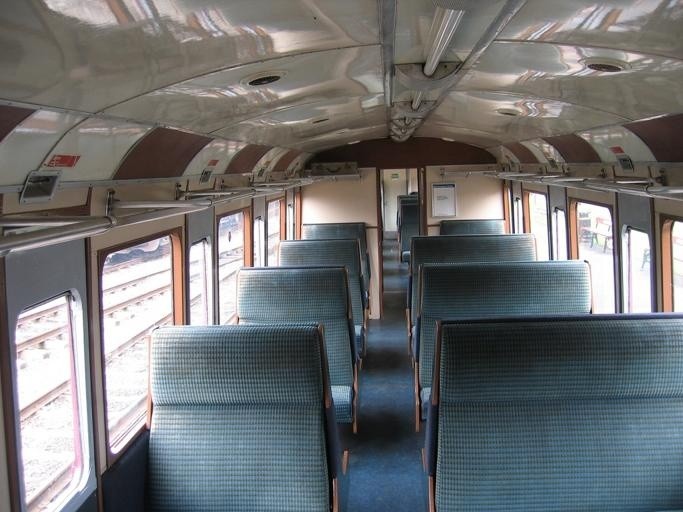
433 183 456 216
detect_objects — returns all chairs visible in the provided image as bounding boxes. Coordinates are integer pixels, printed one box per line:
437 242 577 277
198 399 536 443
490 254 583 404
395 194 418 263
148 217 372 511
406 220 683 511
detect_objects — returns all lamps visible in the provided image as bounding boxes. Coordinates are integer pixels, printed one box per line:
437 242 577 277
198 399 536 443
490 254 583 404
388 0 498 143
